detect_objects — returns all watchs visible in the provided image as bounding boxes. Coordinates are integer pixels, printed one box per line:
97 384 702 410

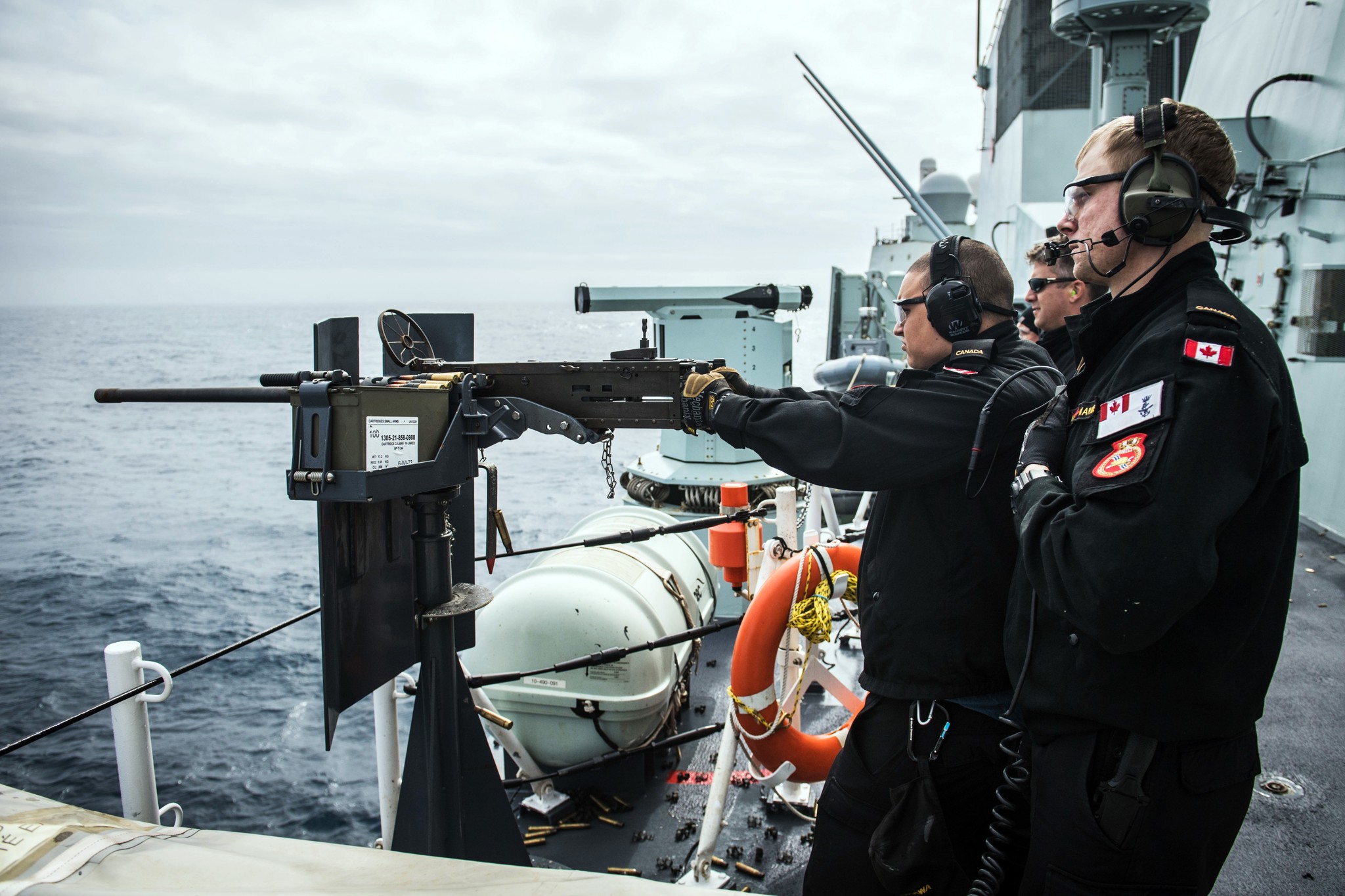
1009 470 1062 516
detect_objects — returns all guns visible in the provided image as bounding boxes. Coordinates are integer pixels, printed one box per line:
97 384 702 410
84 352 734 441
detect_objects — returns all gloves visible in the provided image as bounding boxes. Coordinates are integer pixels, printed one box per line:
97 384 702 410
681 372 734 436
710 366 754 398
1016 394 1069 479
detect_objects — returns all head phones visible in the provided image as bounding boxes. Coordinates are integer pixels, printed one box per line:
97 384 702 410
1117 104 1202 247
921 235 986 342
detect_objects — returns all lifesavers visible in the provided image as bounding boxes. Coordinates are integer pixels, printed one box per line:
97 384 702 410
734 548 886 797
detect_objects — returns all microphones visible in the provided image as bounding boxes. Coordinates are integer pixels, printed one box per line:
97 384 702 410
1043 225 1134 267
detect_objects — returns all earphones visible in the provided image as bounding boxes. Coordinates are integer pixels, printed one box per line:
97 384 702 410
1071 291 1076 296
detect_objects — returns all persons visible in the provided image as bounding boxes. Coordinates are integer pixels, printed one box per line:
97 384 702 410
682 97 1310 896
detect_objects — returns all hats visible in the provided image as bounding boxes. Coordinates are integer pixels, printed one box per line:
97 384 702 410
1017 307 1042 337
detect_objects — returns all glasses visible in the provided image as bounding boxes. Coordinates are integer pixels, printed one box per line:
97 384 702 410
1028 278 1090 292
892 296 925 326
1062 171 1128 222
